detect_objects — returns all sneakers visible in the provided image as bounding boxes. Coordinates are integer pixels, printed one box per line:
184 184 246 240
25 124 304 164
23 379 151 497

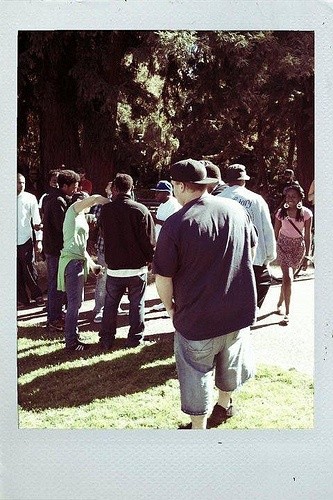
65 342 90 351
115 310 129 315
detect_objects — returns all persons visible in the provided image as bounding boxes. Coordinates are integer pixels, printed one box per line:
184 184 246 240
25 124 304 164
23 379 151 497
200 159 315 326
16 168 111 351
87 172 184 348
151 158 260 429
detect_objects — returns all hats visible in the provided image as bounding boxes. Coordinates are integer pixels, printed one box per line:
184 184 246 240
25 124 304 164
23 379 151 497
170 158 217 185
225 164 250 181
150 180 172 194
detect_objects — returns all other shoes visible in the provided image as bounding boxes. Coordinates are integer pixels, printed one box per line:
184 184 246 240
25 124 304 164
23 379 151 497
78 334 86 340
45 317 65 332
19 303 35 309
97 342 115 352
277 304 284 315
125 338 139 346
210 397 234 424
282 316 289 325
93 313 102 323
36 301 47 307
